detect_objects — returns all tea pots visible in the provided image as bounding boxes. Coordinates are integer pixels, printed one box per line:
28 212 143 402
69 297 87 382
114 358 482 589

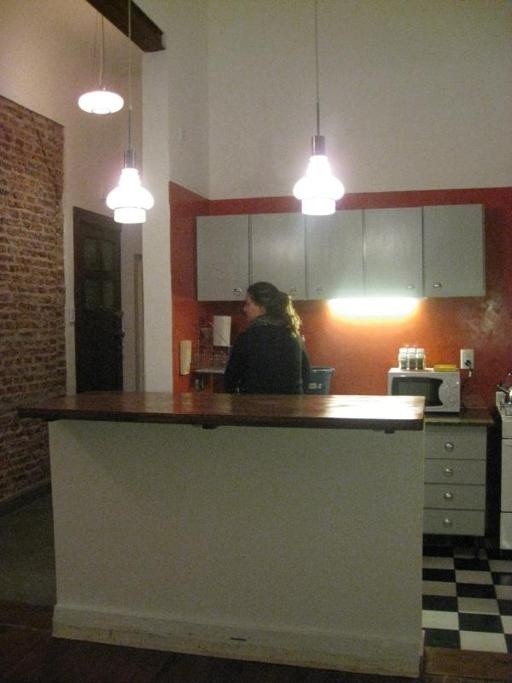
496 372 512 405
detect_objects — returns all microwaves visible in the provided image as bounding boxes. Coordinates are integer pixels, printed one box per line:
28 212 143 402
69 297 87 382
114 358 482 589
387 367 460 413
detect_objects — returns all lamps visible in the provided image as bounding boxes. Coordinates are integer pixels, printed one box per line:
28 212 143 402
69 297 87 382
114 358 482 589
76 14 124 116
104 0 156 226
289 0 344 217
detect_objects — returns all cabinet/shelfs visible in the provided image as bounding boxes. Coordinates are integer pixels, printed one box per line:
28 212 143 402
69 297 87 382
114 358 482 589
193 203 488 302
499 422 512 550
422 422 497 537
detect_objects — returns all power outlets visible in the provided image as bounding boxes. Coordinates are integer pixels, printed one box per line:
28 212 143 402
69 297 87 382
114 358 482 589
459 349 475 370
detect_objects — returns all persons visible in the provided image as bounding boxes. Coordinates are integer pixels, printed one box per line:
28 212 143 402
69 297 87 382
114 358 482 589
220 280 312 397
